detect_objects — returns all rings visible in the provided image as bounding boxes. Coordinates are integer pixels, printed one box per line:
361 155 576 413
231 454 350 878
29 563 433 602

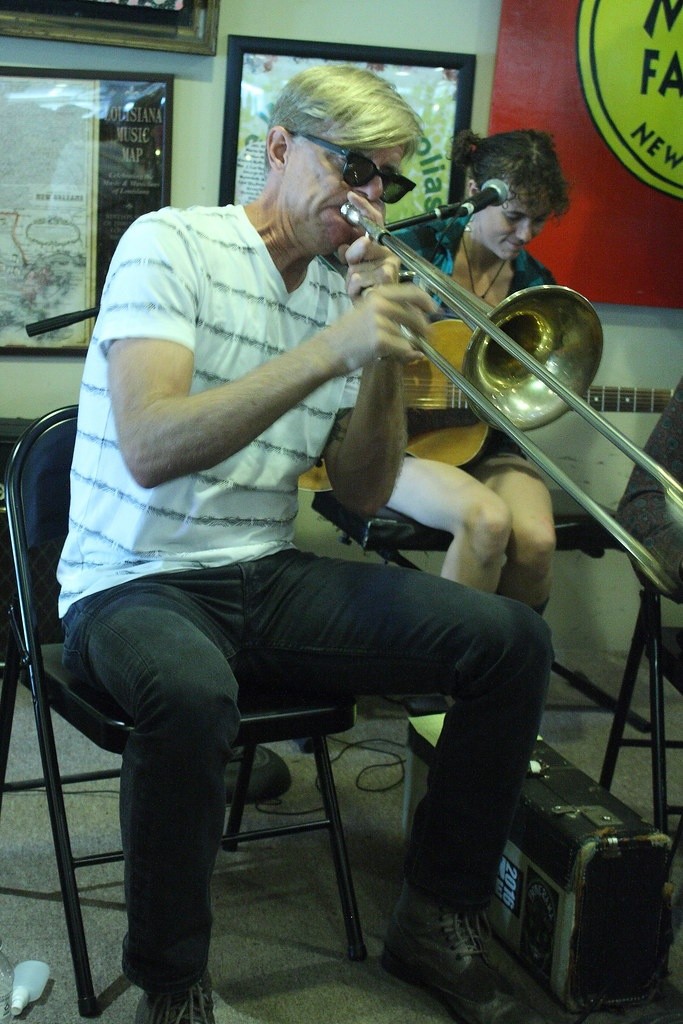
363 286 378 301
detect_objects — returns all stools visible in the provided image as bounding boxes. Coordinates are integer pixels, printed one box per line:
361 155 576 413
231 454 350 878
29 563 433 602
310 482 672 841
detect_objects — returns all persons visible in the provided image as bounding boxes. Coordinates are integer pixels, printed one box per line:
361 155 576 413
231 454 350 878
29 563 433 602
614 376 683 605
56 64 554 1024
386 129 573 618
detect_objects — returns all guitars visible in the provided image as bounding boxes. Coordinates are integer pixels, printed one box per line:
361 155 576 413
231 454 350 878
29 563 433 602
298 317 676 493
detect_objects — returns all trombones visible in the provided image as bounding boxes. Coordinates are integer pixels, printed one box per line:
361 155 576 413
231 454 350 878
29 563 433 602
336 202 683 614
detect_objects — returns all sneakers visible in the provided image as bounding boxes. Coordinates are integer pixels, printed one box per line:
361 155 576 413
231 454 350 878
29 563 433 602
380 877 542 1024
136 970 215 1024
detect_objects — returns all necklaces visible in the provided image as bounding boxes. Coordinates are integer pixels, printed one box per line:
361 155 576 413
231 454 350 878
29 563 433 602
459 235 507 302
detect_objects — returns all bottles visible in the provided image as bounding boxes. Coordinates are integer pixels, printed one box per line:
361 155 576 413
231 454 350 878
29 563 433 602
0 938 14 1024
11 960 50 1015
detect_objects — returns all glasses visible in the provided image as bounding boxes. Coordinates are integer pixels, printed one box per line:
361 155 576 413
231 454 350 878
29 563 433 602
285 127 416 204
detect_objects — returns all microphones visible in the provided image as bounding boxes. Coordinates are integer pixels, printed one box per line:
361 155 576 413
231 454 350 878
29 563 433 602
456 177 511 217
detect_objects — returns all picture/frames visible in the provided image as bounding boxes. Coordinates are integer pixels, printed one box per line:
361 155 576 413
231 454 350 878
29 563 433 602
214 28 481 216
0 0 221 58
0 64 179 363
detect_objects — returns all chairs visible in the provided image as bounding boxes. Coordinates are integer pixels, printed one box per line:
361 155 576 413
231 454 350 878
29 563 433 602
0 400 372 1019
